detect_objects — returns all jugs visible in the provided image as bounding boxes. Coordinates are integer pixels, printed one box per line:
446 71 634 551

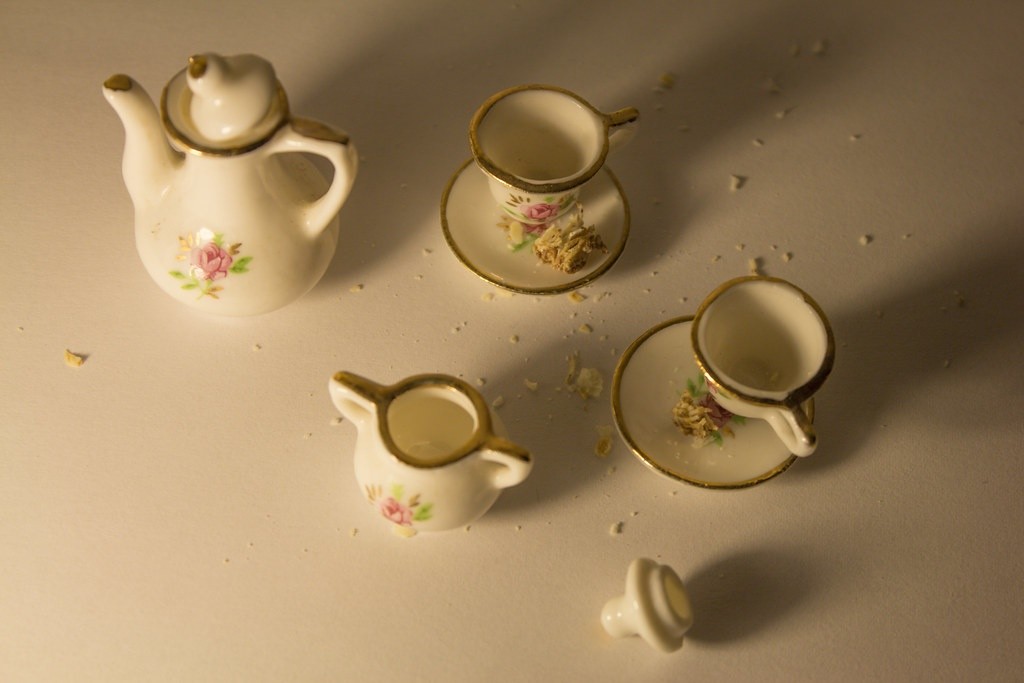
98 51 359 320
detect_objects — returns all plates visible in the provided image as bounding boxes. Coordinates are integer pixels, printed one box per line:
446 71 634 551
608 317 805 496
438 160 632 298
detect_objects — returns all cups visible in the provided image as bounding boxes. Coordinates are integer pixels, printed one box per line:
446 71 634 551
468 84 640 228
691 275 836 459
322 370 534 535
